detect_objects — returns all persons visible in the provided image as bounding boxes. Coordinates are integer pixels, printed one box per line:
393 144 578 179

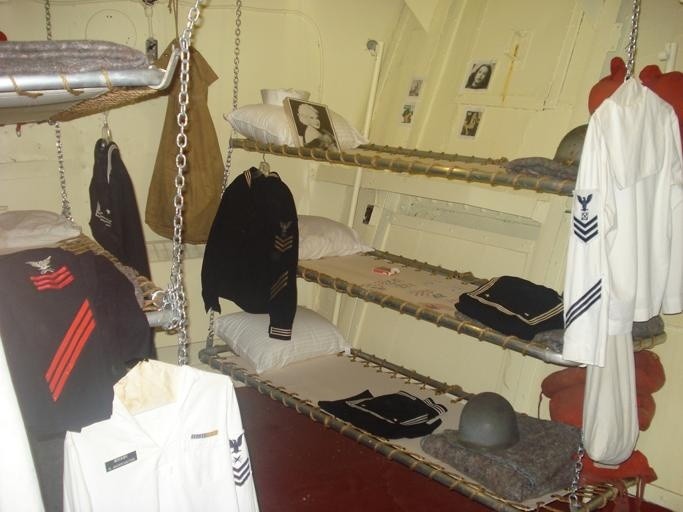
462 109 479 134
466 63 491 88
297 103 331 149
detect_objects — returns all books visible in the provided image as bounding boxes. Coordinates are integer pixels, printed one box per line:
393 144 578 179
282 97 342 153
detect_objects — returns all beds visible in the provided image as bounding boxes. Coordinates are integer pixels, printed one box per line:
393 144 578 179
0 49 180 127
289 245 667 367
229 131 579 198
199 340 654 512
43 224 186 331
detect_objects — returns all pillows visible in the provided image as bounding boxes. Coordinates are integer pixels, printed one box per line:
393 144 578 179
2 207 82 249
294 214 374 261
223 103 368 150
211 303 351 376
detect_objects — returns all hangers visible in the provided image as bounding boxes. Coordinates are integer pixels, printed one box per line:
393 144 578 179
97 113 112 147
255 148 271 175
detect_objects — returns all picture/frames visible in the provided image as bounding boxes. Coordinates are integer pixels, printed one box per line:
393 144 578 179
282 96 340 152
